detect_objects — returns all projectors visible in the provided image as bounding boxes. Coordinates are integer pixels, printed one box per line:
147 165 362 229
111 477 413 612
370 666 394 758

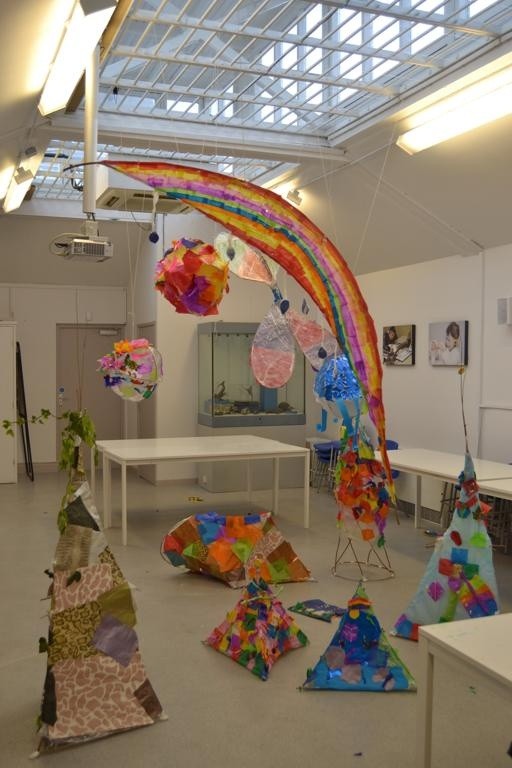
63 238 113 263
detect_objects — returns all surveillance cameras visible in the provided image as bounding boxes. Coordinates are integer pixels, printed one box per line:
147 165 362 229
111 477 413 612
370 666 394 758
149 231 159 243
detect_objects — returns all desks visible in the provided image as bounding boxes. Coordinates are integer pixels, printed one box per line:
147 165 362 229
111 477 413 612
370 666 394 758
415 610 512 767
371 447 511 554
90 435 310 542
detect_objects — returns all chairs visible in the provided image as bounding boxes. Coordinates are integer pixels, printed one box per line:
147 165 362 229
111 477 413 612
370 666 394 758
363 438 410 523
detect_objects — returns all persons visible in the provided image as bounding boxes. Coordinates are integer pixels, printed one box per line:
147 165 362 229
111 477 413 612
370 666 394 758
384 326 397 348
428 322 462 366
